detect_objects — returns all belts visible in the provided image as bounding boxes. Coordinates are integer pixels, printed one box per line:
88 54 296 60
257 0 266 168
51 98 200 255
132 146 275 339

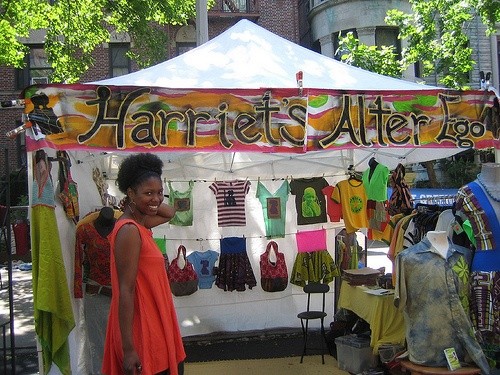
85 284 113 297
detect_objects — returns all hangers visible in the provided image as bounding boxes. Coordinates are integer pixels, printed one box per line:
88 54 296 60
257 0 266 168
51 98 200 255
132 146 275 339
409 195 459 230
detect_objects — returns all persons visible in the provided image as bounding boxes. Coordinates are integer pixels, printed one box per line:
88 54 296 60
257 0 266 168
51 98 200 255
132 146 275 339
363 158 390 230
106 151 188 375
451 161 500 375
397 230 476 371
74 206 131 375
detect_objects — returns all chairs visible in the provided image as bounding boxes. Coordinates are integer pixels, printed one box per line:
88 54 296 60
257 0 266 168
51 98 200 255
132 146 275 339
297 283 330 365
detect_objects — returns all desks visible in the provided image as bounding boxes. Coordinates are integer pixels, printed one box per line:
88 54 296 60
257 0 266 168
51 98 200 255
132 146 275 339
338 281 407 350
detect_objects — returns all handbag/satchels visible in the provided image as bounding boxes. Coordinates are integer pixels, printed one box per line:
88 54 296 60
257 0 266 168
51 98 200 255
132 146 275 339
168 245 199 296
261 241 288 292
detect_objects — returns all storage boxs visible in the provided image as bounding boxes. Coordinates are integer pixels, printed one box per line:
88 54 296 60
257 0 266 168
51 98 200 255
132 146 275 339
335 334 378 374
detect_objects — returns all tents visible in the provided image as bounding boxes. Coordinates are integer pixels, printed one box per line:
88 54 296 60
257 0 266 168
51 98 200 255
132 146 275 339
23 17 497 372
